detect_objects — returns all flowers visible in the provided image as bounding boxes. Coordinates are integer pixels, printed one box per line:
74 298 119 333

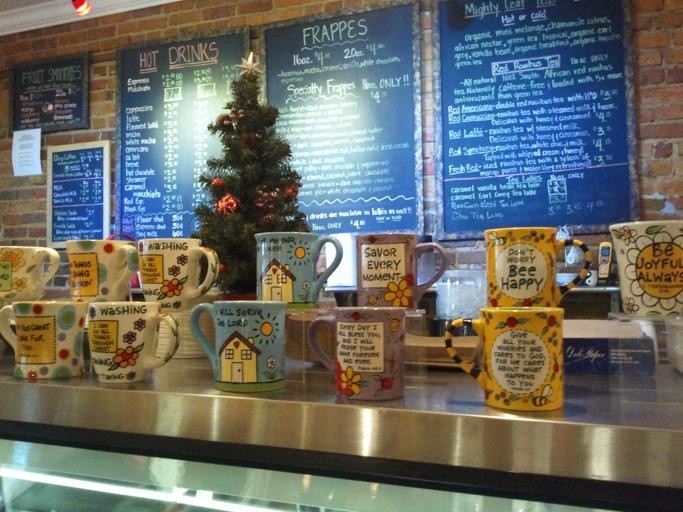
107 317 147 381
336 351 398 397
0 247 28 292
489 292 561 382
343 312 372 320
157 254 188 300
298 280 311 302
263 355 279 379
612 225 683 316
384 273 414 308
89 306 96 319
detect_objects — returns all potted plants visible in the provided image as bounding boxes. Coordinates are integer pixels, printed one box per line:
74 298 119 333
195 51 312 300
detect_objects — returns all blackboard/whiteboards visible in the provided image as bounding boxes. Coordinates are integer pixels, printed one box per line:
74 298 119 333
259 0 426 245
47 140 109 249
9 53 89 133
430 0 641 241
115 26 252 244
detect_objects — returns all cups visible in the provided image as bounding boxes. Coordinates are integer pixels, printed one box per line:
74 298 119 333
187 300 287 394
445 306 564 409
356 233 448 309
136 238 220 313
254 232 344 309
306 306 404 400
607 219 682 319
0 301 86 380
0 246 60 308
88 302 182 385
485 226 590 307
65 240 139 302
